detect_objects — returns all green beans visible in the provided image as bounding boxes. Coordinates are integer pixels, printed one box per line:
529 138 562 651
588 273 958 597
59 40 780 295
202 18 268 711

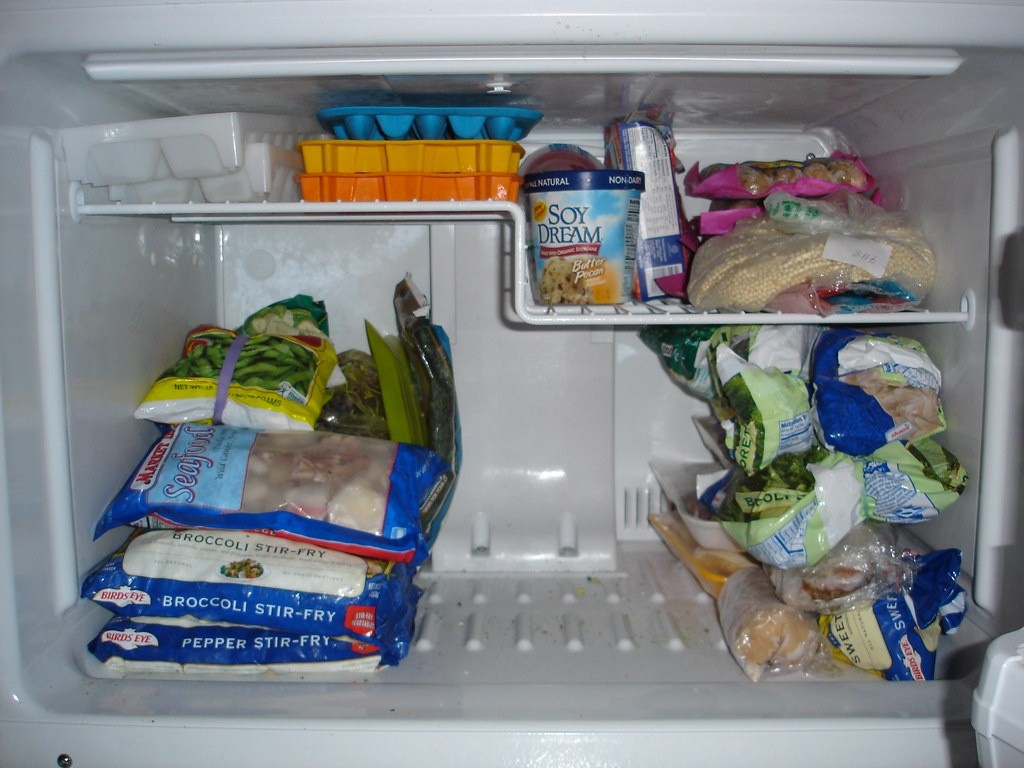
154 331 316 400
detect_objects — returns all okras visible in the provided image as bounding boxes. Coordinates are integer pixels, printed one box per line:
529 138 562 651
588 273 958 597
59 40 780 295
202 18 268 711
363 319 425 446
